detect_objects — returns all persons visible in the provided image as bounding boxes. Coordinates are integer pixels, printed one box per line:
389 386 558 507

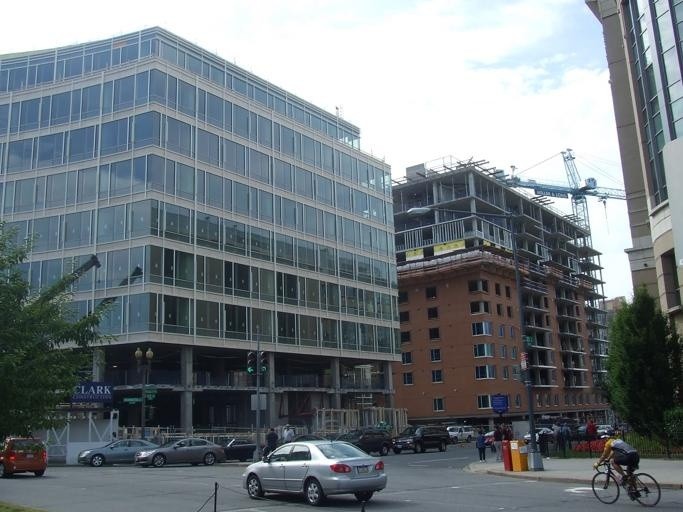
593 427 641 501
265 428 278 452
586 420 596 436
282 424 294 444
475 426 512 463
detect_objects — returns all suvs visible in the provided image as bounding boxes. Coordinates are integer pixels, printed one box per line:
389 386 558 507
392 425 450 454
0 437 46 478
446 425 474 443
335 429 391 457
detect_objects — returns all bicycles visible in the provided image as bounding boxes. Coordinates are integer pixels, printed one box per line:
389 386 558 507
591 459 660 507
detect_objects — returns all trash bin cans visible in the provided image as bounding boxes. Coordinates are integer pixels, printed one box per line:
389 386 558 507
527 451 544 471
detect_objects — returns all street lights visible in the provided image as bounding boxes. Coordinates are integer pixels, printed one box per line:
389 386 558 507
406 207 545 473
134 346 153 439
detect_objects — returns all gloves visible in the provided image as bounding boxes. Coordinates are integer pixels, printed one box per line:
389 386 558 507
591 463 598 469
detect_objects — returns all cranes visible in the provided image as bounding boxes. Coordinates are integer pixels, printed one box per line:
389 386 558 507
504 147 627 369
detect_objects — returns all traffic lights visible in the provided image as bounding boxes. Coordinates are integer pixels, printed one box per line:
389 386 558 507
247 351 255 376
258 350 267 375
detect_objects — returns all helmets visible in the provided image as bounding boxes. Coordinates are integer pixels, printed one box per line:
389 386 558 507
599 430 612 439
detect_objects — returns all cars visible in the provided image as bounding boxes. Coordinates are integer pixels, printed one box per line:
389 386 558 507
241 439 387 505
523 428 554 444
77 439 161 466
276 434 325 450
134 437 227 467
578 423 614 433
223 439 255 461
484 430 495 445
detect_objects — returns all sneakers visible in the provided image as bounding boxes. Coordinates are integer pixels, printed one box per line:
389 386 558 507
630 492 639 501
619 475 627 486
